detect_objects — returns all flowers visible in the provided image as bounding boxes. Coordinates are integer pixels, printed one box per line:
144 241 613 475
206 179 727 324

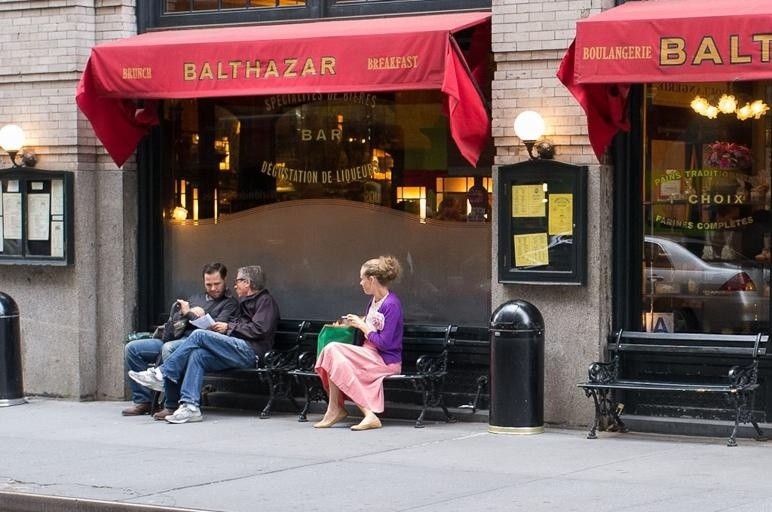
696 140 755 175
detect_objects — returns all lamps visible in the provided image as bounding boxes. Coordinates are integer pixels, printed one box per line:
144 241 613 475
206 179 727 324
513 108 559 161
0 121 41 170
688 81 770 123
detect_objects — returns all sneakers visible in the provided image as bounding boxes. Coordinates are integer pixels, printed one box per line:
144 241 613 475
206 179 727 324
164 402 203 424
127 366 166 393
122 400 153 416
153 408 177 421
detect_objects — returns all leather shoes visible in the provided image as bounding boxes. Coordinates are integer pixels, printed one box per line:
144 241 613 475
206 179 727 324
313 406 349 428
350 416 383 431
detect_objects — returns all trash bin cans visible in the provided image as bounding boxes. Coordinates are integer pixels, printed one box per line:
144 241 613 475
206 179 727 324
487 300 544 434
0 291 28 407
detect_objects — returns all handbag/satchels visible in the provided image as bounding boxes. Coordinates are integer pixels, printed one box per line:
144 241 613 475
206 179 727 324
160 302 188 340
316 322 355 359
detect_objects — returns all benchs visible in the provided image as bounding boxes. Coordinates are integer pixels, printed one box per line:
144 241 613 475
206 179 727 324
275 319 463 428
141 319 312 418
575 326 770 446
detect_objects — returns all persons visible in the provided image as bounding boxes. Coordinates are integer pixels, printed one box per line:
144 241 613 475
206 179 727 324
121 261 237 421
312 254 405 431
127 265 281 423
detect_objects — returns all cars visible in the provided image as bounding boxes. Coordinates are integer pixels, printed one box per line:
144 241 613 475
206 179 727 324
641 233 770 331
